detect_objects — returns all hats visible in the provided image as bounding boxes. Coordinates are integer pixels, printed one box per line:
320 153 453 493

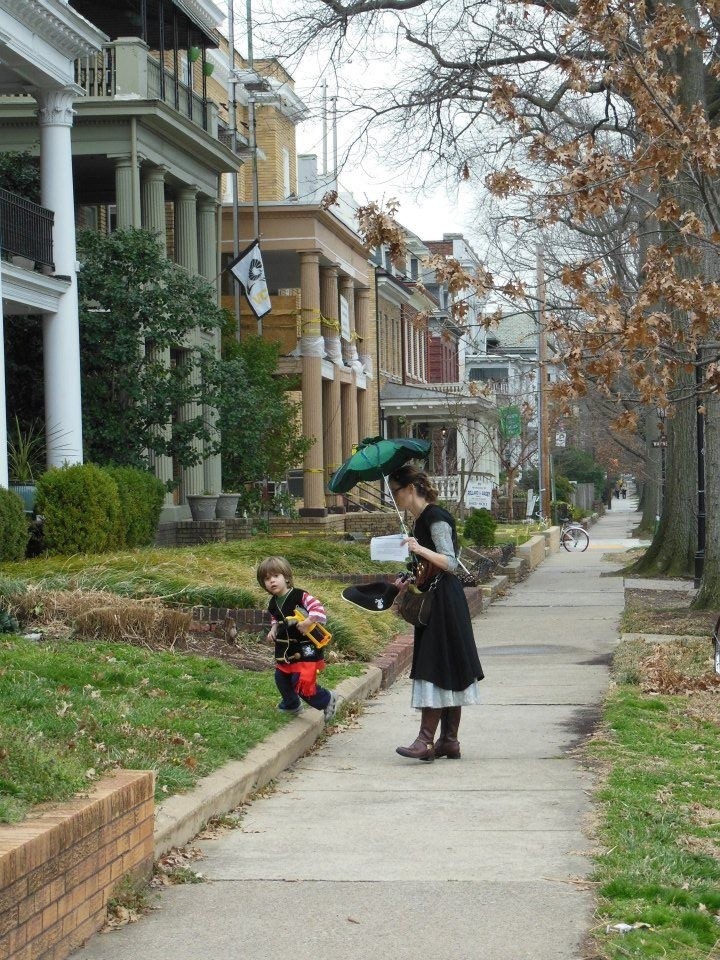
341 582 400 612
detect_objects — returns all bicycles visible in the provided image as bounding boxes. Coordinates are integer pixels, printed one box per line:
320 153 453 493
534 510 590 552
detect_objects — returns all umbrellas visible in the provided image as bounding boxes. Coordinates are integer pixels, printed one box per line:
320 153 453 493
327 437 432 564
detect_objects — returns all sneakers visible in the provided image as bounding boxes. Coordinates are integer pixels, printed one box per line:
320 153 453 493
277 701 304 715
323 690 338 723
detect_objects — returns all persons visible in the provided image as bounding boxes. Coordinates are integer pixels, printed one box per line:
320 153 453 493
616 483 627 499
257 557 335 722
388 466 484 761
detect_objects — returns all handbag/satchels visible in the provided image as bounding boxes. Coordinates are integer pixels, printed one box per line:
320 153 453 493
398 589 435 625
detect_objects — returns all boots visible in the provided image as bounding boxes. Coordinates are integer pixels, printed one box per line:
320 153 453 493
396 706 442 761
433 705 461 759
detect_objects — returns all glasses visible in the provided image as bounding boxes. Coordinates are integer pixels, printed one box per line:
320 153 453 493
391 481 408 496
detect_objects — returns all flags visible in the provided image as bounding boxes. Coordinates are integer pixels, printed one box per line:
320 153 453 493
228 239 271 321
